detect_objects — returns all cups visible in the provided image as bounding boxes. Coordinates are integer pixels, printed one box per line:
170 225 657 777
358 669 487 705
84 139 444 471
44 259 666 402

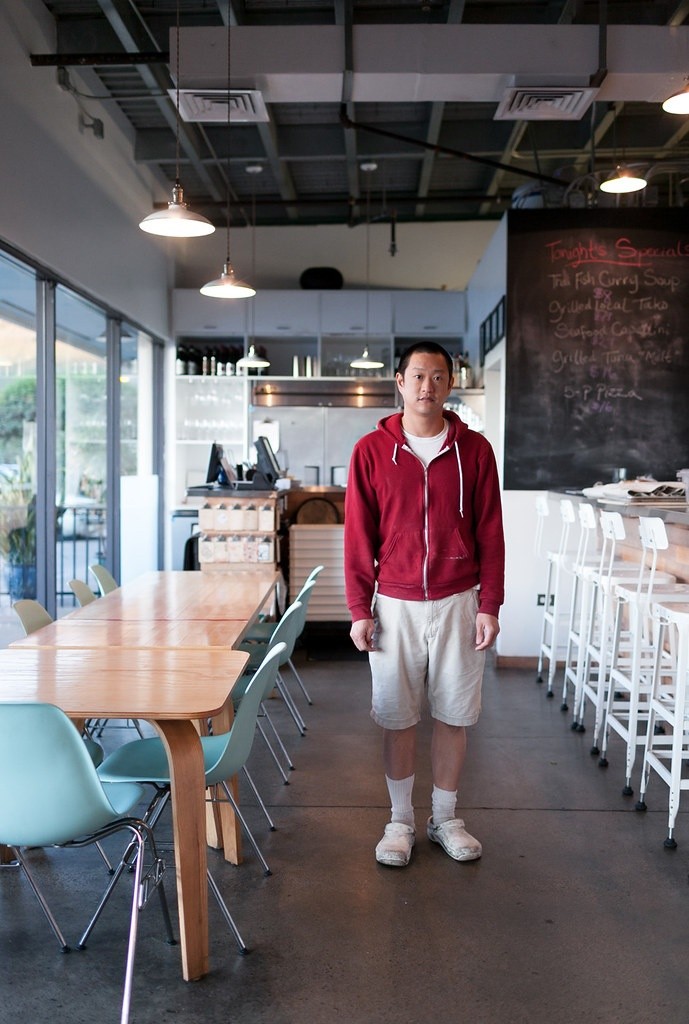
614 468 626 482
217 362 225 375
236 363 243 376
307 355 319 376
226 362 235 375
292 356 305 377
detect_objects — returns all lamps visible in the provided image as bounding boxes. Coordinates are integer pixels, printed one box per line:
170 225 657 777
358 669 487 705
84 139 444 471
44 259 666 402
661 75 689 115
599 99 648 193
350 161 385 369
198 0 257 298
236 164 270 368
138 0 215 238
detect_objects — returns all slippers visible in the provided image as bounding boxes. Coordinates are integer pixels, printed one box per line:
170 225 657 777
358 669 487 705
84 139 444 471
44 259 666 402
375 822 415 866
426 815 483 861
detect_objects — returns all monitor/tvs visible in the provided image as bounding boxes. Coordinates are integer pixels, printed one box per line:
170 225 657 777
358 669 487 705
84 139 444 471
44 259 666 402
206 444 236 489
253 437 281 479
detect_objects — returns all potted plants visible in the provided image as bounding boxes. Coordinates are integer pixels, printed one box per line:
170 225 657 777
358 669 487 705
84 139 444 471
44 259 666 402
7 492 36 600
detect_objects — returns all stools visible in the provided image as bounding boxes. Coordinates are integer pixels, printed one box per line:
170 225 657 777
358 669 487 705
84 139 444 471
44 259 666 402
535 488 689 848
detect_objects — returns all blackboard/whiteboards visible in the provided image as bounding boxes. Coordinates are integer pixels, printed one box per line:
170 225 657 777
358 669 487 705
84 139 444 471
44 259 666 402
504 207 689 491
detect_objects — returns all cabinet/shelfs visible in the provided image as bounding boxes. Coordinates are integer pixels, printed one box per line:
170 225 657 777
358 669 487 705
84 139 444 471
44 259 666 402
172 288 484 481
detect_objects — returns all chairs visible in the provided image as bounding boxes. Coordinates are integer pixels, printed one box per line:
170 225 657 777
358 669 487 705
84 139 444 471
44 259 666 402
207 564 326 785
0 700 177 1024
75 641 289 955
12 563 144 766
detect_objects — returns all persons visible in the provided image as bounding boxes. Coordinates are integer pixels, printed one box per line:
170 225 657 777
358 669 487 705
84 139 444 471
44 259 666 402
343 342 505 866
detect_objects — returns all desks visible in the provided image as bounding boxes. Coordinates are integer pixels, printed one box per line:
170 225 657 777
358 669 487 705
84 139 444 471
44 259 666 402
61 570 279 699
9 619 250 850
0 647 252 982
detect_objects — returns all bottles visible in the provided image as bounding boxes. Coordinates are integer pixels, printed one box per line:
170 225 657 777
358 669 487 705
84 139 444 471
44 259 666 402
394 347 473 388
176 344 243 374
249 346 267 374
